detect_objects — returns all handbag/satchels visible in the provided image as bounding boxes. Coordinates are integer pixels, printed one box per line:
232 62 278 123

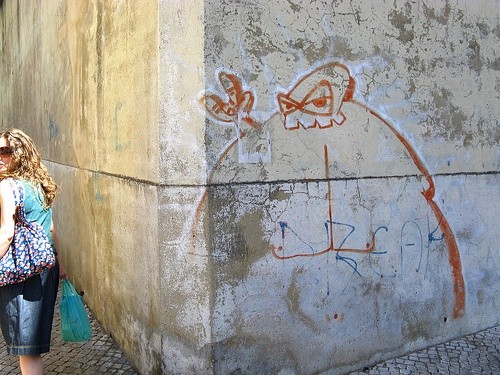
0 177 56 287
59 277 92 342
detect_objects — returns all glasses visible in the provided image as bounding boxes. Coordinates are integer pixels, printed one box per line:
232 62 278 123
0 147 14 158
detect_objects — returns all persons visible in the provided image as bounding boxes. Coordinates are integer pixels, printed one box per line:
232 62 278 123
1 127 67 375
